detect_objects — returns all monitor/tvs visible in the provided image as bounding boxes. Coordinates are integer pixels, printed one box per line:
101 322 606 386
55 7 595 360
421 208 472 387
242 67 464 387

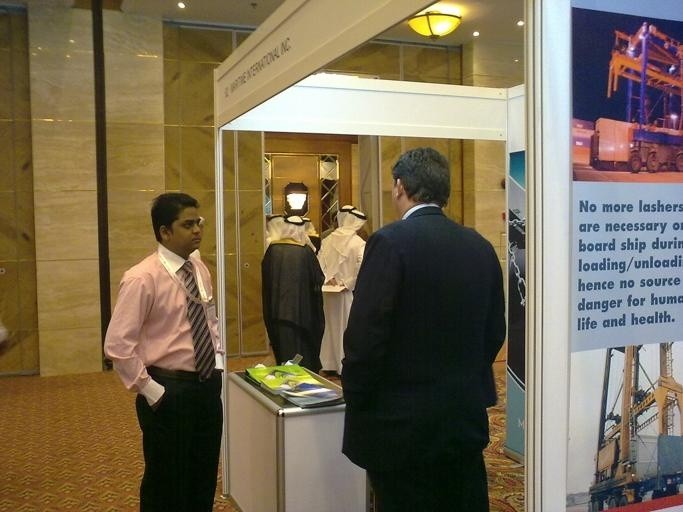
243 364 340 408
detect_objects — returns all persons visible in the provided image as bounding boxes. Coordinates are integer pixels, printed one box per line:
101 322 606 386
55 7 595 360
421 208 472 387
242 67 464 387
343 145 508 510
105 193 222 511
260 205 368 376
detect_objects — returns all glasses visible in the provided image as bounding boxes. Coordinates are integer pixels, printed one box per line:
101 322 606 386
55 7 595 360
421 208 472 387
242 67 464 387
407 11 461 42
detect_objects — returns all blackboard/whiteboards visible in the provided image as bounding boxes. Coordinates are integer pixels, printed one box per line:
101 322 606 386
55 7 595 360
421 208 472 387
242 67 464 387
586 339 682 511
589 21 681 175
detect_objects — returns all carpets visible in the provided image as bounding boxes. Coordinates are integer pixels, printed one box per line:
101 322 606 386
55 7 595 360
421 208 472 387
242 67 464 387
181 262 216 381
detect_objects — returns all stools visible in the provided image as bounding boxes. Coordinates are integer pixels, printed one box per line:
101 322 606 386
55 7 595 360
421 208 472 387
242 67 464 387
149 366 221 383
